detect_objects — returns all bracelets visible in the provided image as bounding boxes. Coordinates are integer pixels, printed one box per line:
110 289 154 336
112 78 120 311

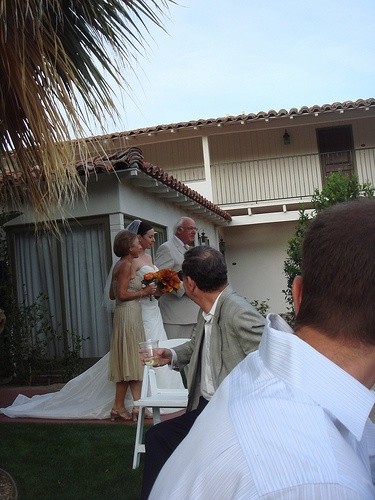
140 288 145 297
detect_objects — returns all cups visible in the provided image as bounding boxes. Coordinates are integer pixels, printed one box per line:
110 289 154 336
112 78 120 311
138 340 159 366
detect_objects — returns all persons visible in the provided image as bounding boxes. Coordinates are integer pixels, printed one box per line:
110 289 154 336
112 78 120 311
109 230 157 421
148 197 375 500
155 217 197 381
0 219 187 420
137 246 266 500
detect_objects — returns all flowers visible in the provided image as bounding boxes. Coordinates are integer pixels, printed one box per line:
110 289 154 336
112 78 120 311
141 268 181 301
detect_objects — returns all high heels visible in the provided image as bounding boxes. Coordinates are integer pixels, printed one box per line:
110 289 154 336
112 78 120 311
110 402 153 421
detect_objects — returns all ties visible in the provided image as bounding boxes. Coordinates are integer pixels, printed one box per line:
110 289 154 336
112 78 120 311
184 244 188 251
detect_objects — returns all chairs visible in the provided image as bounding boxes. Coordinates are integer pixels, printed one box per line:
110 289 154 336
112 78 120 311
133 338 191 469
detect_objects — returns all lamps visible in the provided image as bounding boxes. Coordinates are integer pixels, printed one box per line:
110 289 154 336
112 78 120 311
197 229 209 246
283 128 291 145
